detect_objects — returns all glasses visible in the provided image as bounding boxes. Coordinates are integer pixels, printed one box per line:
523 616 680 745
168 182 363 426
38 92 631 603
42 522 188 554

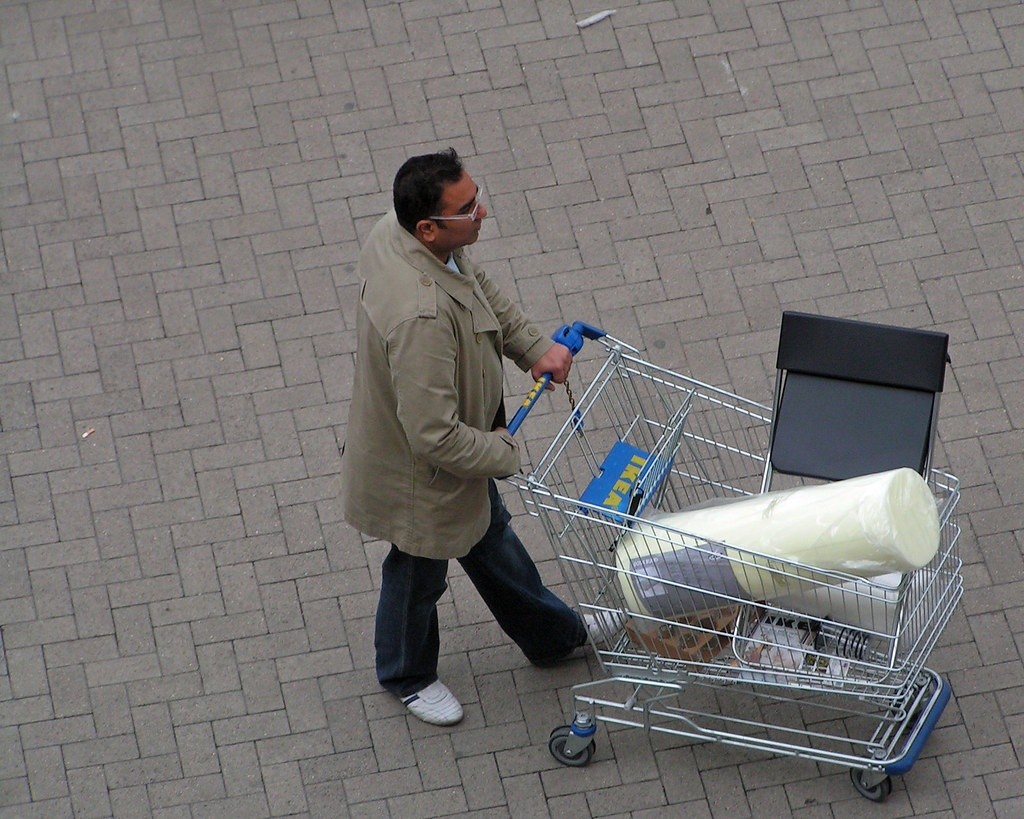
430 184 483 221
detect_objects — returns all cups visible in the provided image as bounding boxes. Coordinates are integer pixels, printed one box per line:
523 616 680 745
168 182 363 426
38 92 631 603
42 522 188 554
813 617 871 662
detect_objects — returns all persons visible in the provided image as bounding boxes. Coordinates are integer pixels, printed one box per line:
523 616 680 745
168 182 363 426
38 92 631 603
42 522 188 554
339 148 631 725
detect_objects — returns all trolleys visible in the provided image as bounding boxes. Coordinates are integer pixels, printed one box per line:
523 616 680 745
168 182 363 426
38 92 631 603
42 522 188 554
495 320 966 803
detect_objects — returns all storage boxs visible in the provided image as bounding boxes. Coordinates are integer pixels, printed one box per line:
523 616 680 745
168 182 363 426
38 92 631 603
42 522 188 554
625 599 752 673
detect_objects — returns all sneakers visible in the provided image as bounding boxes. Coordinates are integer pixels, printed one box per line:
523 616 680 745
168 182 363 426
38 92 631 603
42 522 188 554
400 679 463 727
583 607 629 645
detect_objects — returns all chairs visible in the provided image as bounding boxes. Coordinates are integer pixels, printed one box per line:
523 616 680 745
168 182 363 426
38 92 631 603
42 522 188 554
732 309 953 685
